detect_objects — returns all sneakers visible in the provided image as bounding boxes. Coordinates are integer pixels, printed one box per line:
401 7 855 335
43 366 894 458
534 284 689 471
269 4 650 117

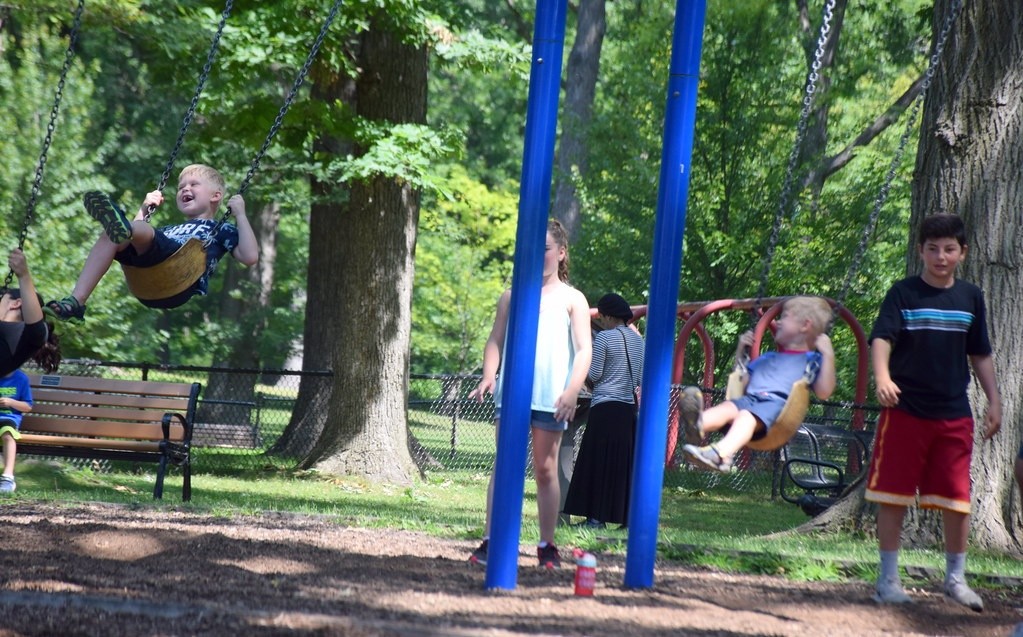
573 517 606 529
469 540 489 566
873 578 912 604
0 474 16 494
684 442 738 476
945 573 984 611
537 543 562 575
679 386 707 443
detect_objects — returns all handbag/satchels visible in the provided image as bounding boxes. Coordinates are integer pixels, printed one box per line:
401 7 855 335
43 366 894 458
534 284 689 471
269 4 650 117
634 386 641 412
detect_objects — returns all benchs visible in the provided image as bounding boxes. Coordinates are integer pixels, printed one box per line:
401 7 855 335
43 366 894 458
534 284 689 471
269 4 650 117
782 424 874 518
17 375 202 502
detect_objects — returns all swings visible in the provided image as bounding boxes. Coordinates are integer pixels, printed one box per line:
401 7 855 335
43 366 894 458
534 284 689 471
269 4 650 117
121 0 343 302
0 0 86 304
721 1 966 455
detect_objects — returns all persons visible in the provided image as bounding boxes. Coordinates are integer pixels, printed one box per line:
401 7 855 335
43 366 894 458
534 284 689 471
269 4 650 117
0 368 34 494
867 216 1001 611
680 296 835 473
0 249 50 382
468 220 593 570
563 293 645 530
42 164 258 326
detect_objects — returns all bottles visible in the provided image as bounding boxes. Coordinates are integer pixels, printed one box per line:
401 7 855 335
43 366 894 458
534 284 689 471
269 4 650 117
573 548 596 596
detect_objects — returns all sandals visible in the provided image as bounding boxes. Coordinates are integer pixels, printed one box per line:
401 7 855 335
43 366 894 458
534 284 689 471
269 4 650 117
82 189 135 245
43 295 86 326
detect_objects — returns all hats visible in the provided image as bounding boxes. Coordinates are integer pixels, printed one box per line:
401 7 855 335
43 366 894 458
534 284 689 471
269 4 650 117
598 294 633 320
